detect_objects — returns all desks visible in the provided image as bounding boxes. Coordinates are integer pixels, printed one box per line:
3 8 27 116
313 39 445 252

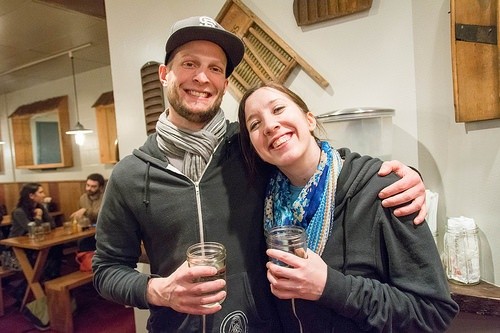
0 211 63 238
0 226 96 311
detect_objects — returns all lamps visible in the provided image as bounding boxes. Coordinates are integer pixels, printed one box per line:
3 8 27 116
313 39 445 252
65 51 92 145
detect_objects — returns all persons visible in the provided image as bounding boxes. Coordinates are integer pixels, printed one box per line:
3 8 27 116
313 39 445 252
91 17 427 333
6 184 60 303
71 174 105 251
238 83 460 333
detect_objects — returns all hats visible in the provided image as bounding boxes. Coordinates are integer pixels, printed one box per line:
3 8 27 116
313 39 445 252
165 16 245 78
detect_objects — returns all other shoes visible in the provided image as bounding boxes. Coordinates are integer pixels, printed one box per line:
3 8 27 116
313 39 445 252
10 286 25 301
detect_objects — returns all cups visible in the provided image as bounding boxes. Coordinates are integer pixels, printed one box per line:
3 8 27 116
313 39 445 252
265 225 307 279
186 242 227 306
64 218 90 235
34 223 51 239
443 225 482 285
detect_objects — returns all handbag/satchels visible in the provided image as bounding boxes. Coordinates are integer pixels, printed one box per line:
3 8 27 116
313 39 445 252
76 251 95 271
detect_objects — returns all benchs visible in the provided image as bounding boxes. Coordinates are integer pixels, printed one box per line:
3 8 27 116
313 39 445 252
43 271 93 333
0 246 78 317
